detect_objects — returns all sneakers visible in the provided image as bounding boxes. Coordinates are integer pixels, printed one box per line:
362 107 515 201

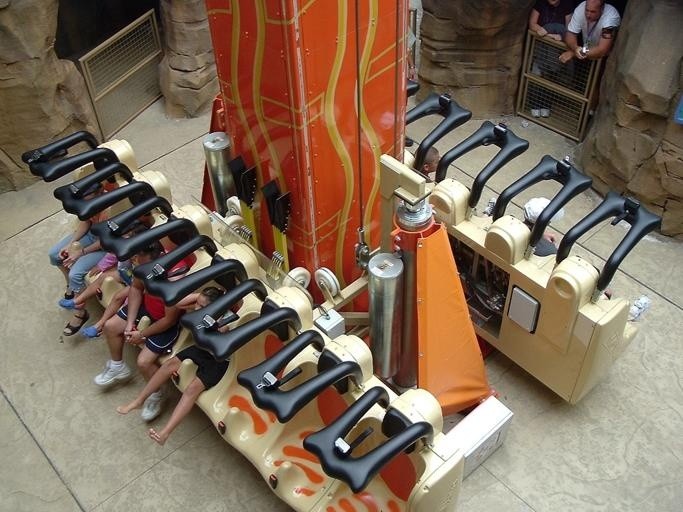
141 384 175 422
541 109 550 117
483 197 496 216
531 109 540 118
94 361 132 386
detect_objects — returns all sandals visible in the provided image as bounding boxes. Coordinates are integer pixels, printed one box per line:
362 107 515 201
63 308 90 337
60 285 86 308
81 325 103 337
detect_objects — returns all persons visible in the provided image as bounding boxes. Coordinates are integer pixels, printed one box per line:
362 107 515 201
50 181 234 447
404 25 418 147
518 196 613 300
528 0 576 118
416 142 496 222
560 0 621 124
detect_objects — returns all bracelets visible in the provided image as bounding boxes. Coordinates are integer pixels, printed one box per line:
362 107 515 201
409 66 415 69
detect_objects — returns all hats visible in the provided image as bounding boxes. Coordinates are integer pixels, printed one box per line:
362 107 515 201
524 197 564 225
